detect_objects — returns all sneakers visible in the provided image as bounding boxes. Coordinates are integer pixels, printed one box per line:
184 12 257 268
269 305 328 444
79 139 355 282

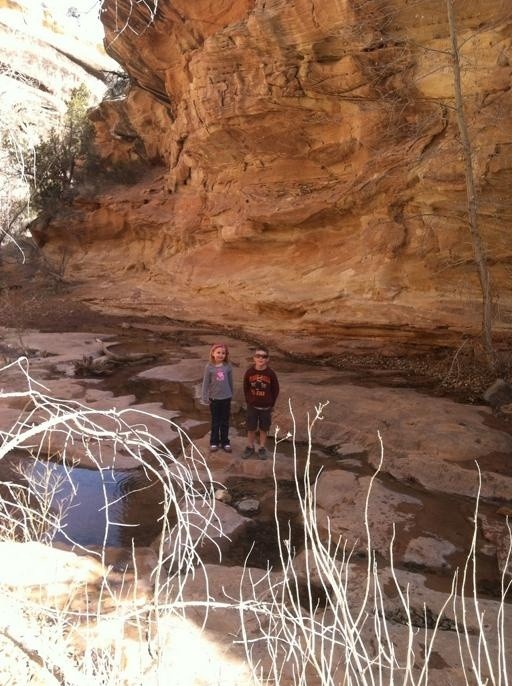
241 445 255 460
257 449 268 461
221 444 232 452
209 445 219 451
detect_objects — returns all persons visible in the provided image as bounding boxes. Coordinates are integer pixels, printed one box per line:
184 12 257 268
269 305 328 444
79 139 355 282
201 344 235 453
241 345 279 461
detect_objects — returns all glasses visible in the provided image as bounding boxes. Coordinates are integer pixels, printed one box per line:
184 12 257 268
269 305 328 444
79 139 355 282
254 354 269 359
